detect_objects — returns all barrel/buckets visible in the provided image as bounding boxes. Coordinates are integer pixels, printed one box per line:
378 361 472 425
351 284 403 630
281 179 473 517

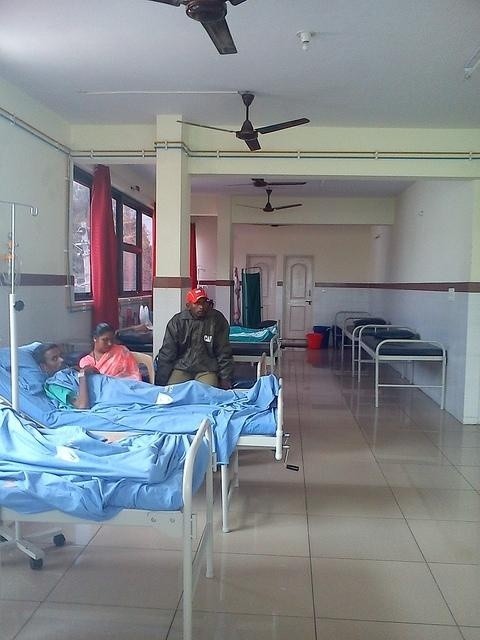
306 333 323 349
313 326 331 349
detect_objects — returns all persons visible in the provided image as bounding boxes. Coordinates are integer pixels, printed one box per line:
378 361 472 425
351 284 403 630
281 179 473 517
33 341 100 409
155 287 234 389
78 322 142 381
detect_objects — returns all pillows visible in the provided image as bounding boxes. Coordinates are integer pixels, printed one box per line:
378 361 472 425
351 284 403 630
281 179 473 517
0 340 69 395
375 330 414 339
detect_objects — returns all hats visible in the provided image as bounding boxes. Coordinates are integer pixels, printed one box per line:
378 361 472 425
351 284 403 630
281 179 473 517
186 287 209 303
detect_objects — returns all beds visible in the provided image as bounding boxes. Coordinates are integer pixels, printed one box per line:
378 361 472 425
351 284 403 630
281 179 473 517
0 365 299 532
334 311 404 379
115 319 286 390
357 325 447 410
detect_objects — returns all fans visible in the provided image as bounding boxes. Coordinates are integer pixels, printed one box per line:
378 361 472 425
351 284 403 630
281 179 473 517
176 94 310 151
226 178 307 227
151 0 246 55
